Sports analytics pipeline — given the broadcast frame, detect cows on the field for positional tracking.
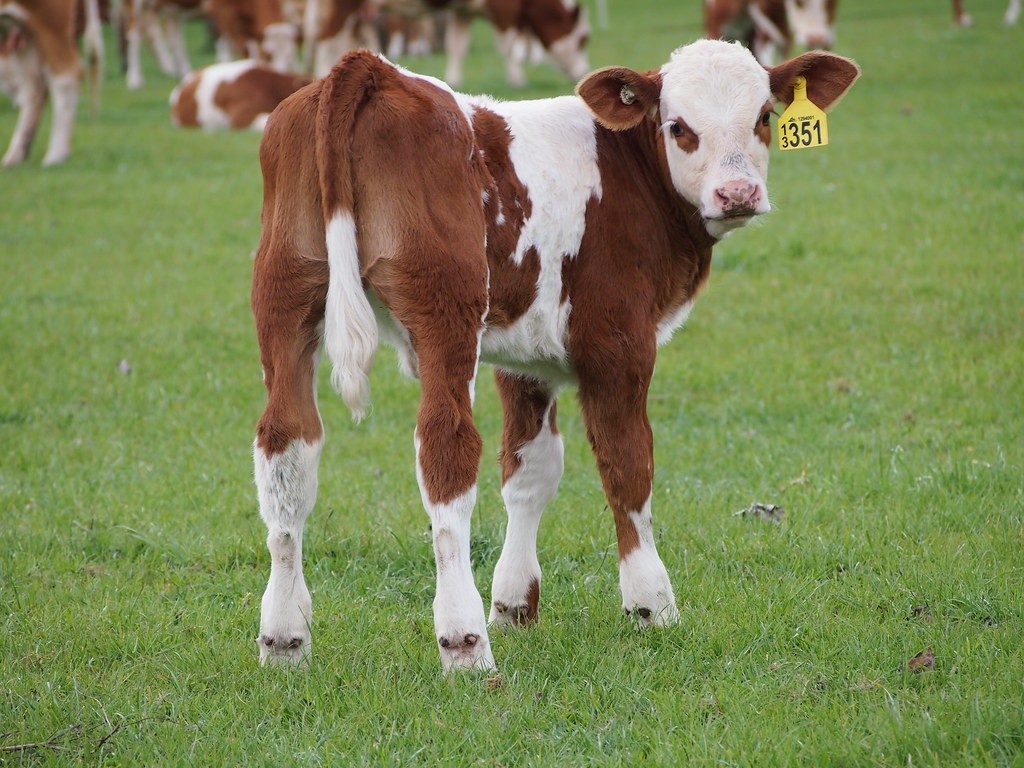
[695,0,841,68]
[251,35,859,690]
[0,1,596,165]
[953,1,1023,29]
